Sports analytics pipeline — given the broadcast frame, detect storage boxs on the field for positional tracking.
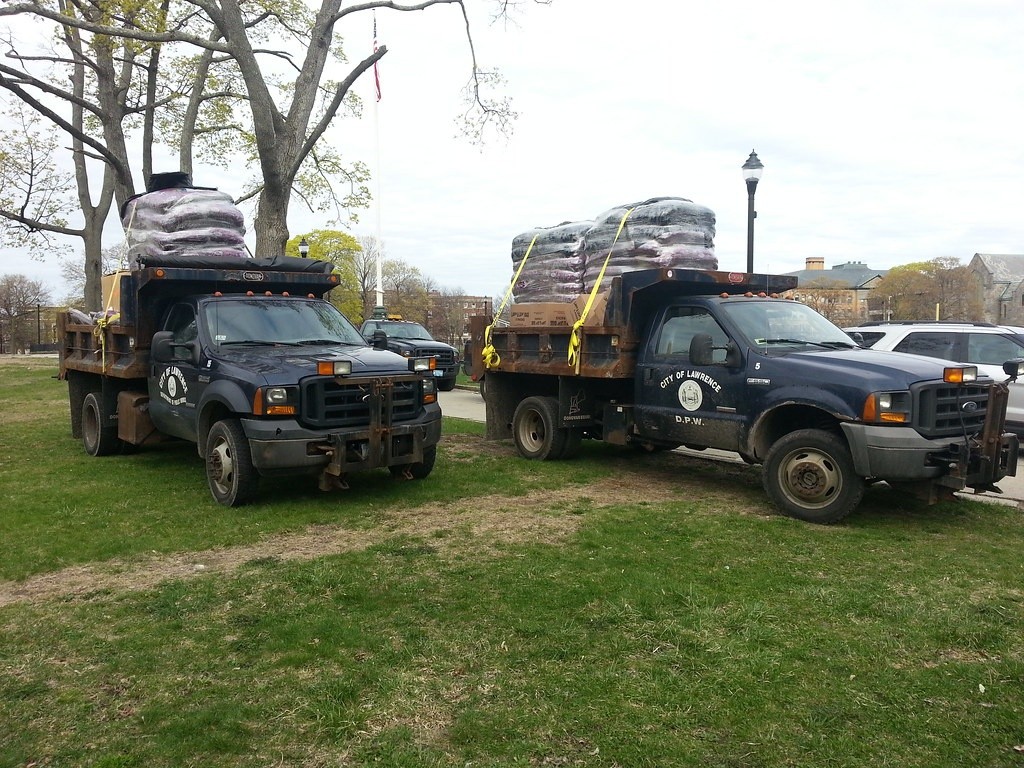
[511,304,572,327]
[112,269,131,312]
[102,272,115,311]
[594,286,612,325]
[564,294,603,325]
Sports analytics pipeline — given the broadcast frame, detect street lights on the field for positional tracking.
[741,148,765,272]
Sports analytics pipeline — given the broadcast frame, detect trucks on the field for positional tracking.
[458,268,1020,526]
[56,264,443,508]
[359,315,461,391]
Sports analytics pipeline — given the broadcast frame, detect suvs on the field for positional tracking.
[839,318,1023,445]
